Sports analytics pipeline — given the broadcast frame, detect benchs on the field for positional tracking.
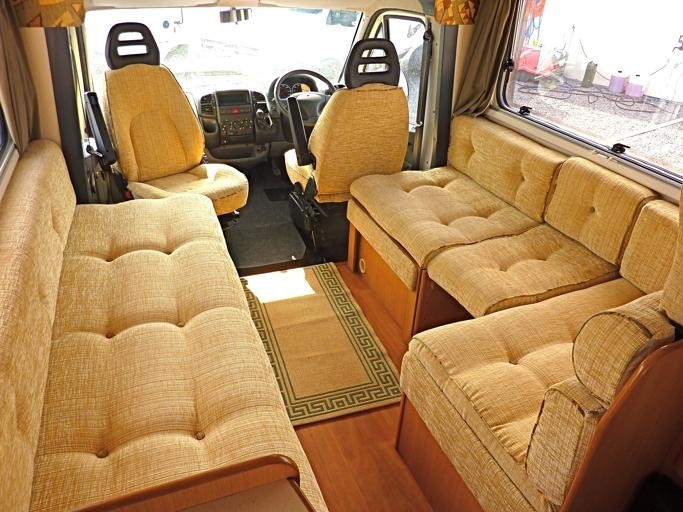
[344,115,660,369]
[0,138,327,512]
[393,191,683,511]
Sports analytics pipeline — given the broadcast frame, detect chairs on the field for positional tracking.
[81,22,249,214]
[284,38,409,256]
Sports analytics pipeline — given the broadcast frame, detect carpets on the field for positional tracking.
[238,261,404,427]
